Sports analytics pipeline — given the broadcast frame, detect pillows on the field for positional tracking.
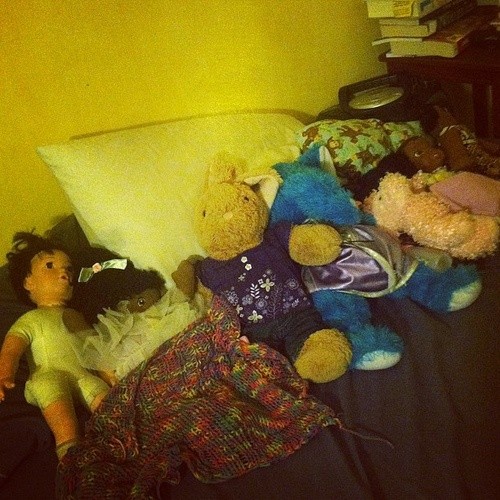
[34,112,309,293]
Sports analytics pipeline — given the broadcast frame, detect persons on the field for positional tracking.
[395,136,500,216]
[0,231,119,461]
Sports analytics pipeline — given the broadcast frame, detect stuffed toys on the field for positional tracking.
[68,144,500,384]
[420,103,500,180]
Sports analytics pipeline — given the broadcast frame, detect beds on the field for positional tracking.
[0,152,500,500]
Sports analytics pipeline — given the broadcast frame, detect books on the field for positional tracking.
[367,0,500,58]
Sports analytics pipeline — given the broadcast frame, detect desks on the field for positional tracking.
[379,5,500,139]
